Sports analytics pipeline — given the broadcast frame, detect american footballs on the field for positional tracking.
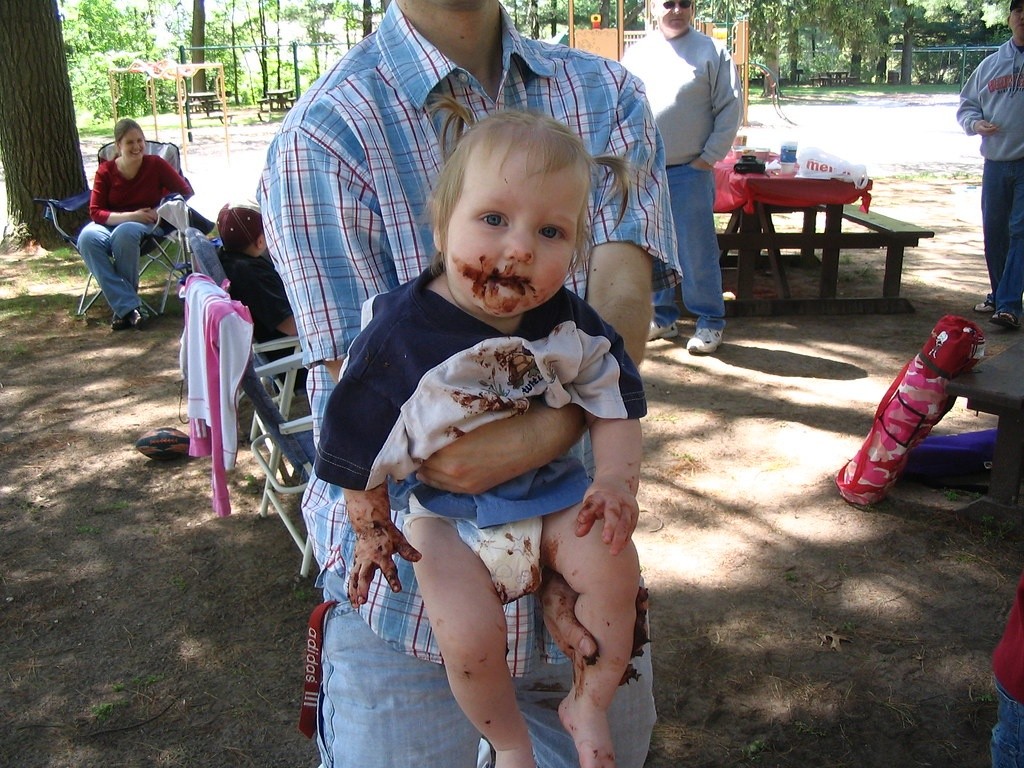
[136,427,190,461]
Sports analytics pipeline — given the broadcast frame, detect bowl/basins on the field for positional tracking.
[731,146,770,161]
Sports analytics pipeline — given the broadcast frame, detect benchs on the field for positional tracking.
[257,96,298,103]
[172,100,226,106]
[809,76,861,80]
[820,203,935,296]
[945,340,1024,505]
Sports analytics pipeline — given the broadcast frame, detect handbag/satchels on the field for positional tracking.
[793,147,868,189]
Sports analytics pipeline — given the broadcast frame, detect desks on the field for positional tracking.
[187,91,222,117]
[694,148,873,317]
[266,89,294,112]
[826,71,850,86]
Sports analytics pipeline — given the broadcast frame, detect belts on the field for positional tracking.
[666,164,688,170]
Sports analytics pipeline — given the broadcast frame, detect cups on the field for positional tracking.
[781,142,797,161]
[779,161,796,176]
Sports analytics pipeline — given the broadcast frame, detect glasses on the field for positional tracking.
[662,0,692,10]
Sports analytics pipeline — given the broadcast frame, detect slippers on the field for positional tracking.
[988,311,1022,328]
[973,301,996,312]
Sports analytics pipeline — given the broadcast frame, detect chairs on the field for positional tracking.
[181,228,298,441]
[32,140,198,317]
[180,274,320,577]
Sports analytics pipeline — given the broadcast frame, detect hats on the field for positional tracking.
[217,201,263,251]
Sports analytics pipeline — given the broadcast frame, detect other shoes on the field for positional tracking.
[110,317,126,330]
[128,307,150,329]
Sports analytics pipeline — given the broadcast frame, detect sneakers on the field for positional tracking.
[687,328,724,354]
[645,321,678,341]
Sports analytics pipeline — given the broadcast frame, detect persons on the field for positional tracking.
[78,118,193,331]
[990,569,1024,768]
[217,199,310,391]
[315,101,653,768]
[621,0,744,356]
[258,0,684,768]
[956,0,1024,330]
